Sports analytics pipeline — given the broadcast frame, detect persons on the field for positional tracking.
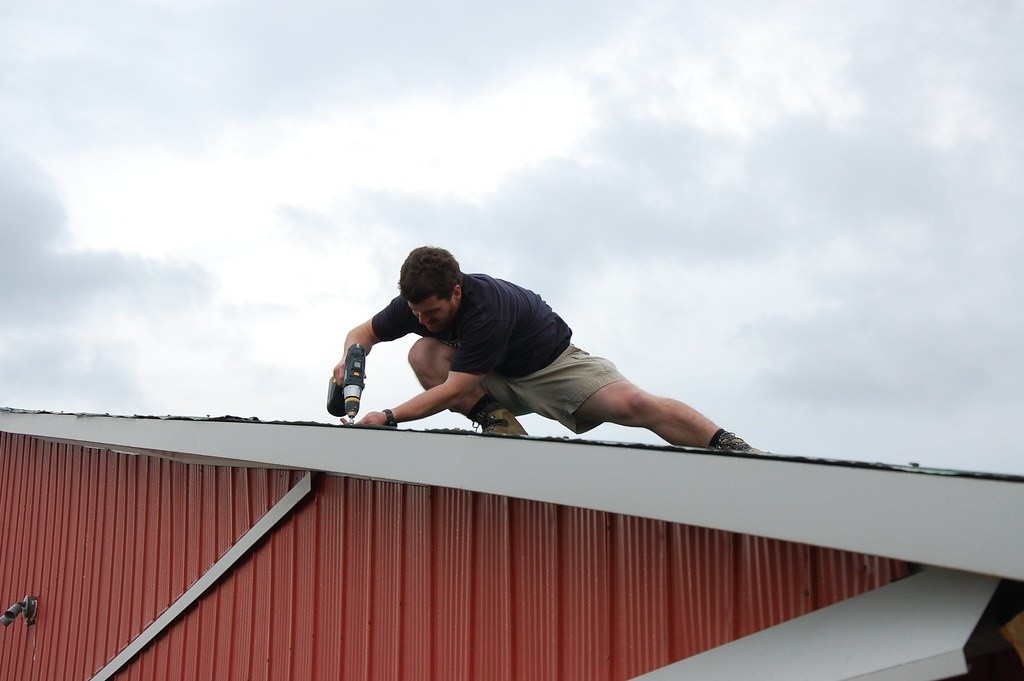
[335,247,765,455]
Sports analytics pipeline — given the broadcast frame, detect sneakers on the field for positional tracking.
[714,431,772,455]
[472,405,528,436]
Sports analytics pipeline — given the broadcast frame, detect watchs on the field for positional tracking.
[382,409,398,428]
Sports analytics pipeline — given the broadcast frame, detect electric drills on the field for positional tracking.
[326,342,367,427]
